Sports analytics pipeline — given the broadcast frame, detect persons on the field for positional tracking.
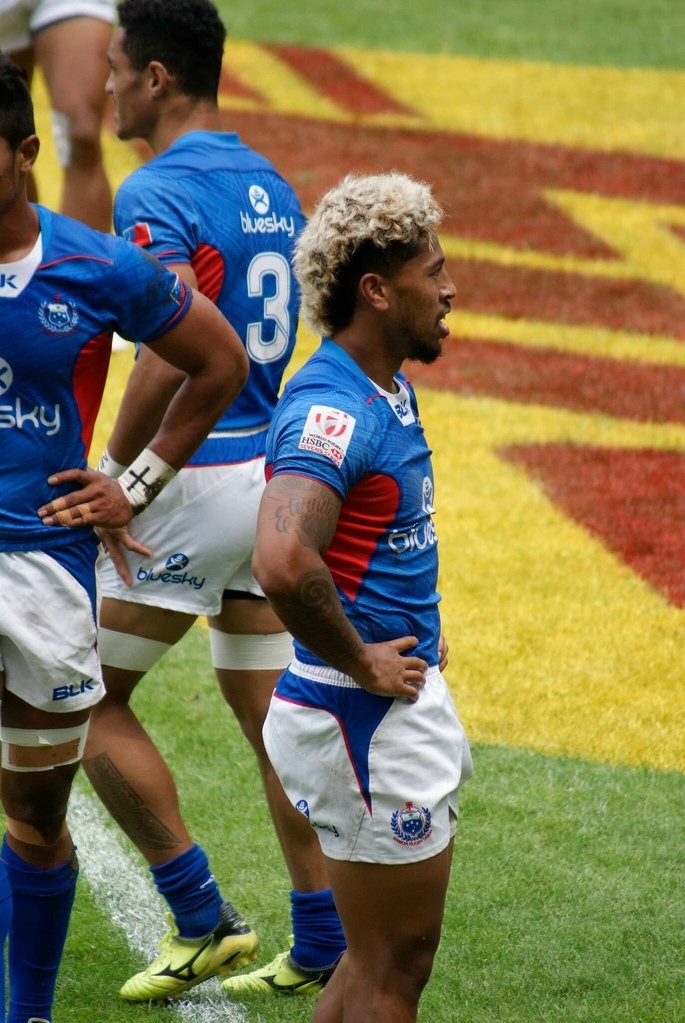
[0,56,251,1022]
[82,0,348,1004]
[0,0,123,234]
[251,170,475,1023]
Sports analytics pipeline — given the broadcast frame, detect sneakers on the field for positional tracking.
[221,932,325,994]
[119,900,258,1000]
